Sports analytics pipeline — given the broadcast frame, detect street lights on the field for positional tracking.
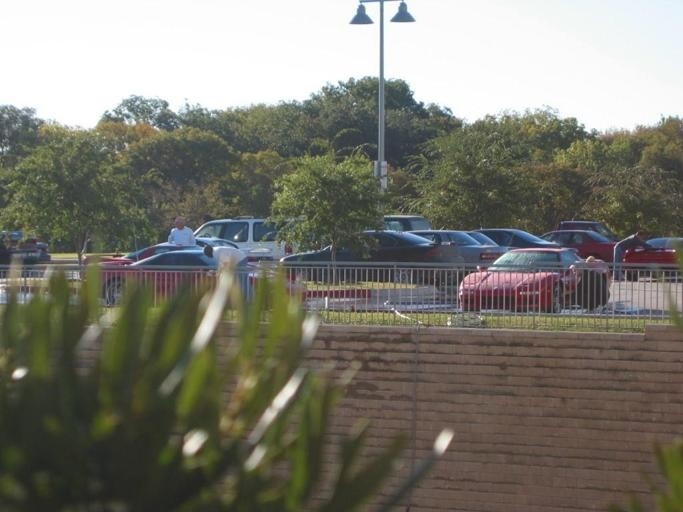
[350,0,415,190]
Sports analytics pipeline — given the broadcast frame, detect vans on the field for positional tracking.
[194,216,329,262]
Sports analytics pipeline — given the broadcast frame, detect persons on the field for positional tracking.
[570,255,611,287]
[168,217,196,248]
[612,229,655,281]
[0,229,50,280]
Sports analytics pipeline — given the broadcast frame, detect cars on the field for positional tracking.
[623,237,682,282]
[82,238,307,310]
[0,230,49,275]
